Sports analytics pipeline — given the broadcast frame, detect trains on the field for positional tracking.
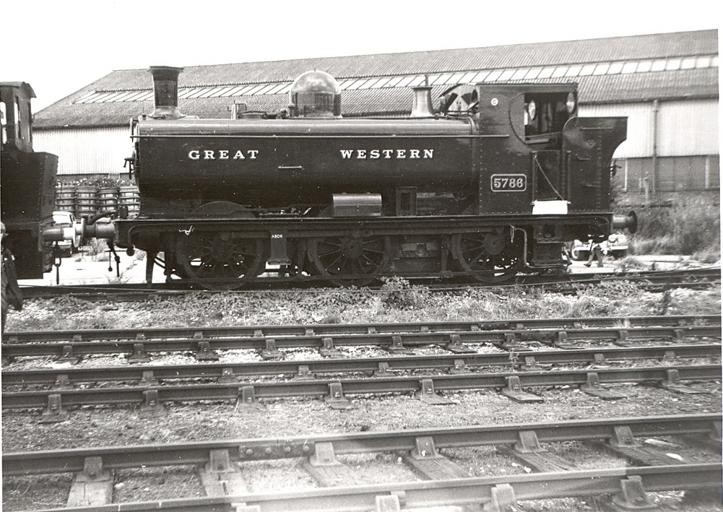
[0,54,643,294]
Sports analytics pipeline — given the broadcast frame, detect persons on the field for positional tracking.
[0,222,23,366]
[583,240,605,267]
